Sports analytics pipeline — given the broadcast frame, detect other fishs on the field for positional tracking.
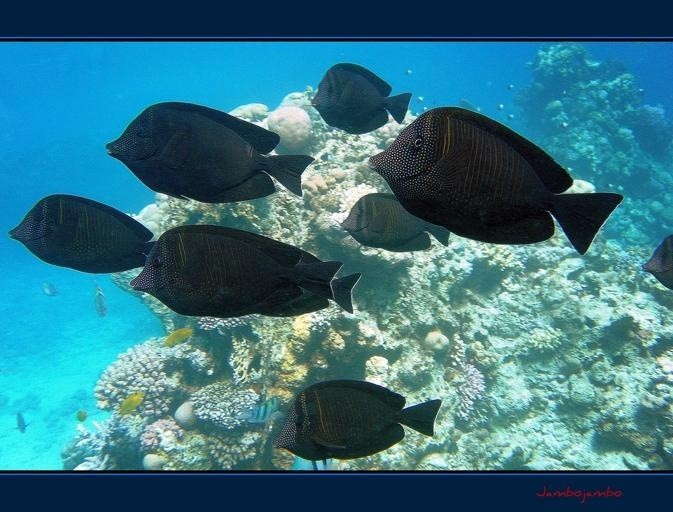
[368,97,625,256]
